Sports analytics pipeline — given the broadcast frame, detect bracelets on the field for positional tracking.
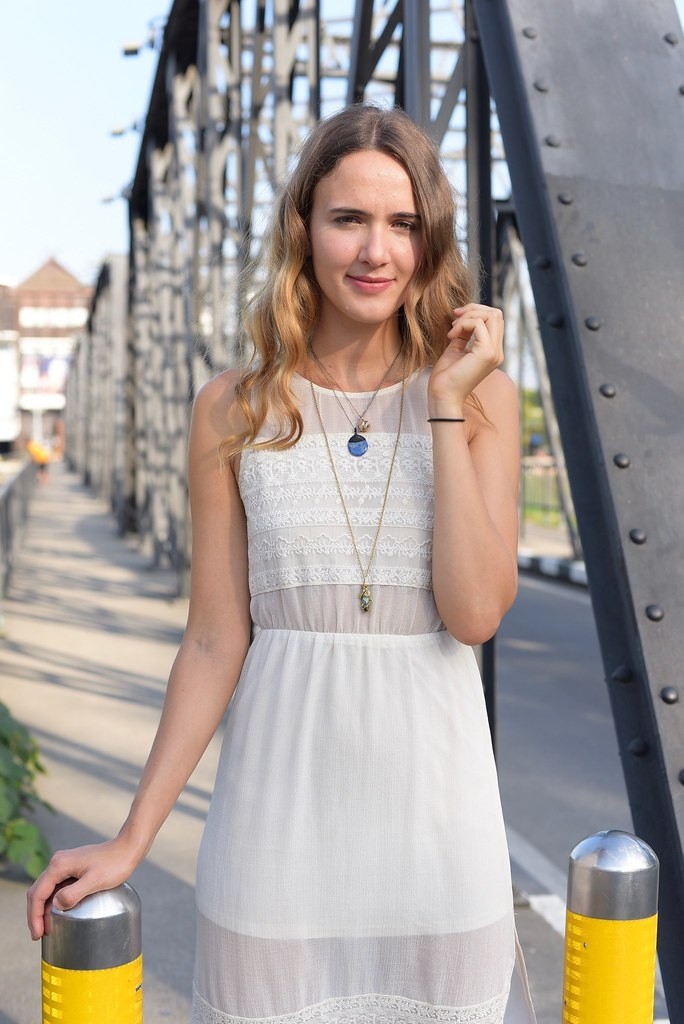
[427,417,465,422]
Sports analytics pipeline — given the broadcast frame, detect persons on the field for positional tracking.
[22,435,51,485]
[27,107,519,1024]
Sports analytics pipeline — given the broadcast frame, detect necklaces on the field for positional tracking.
[308,340,409,613]
